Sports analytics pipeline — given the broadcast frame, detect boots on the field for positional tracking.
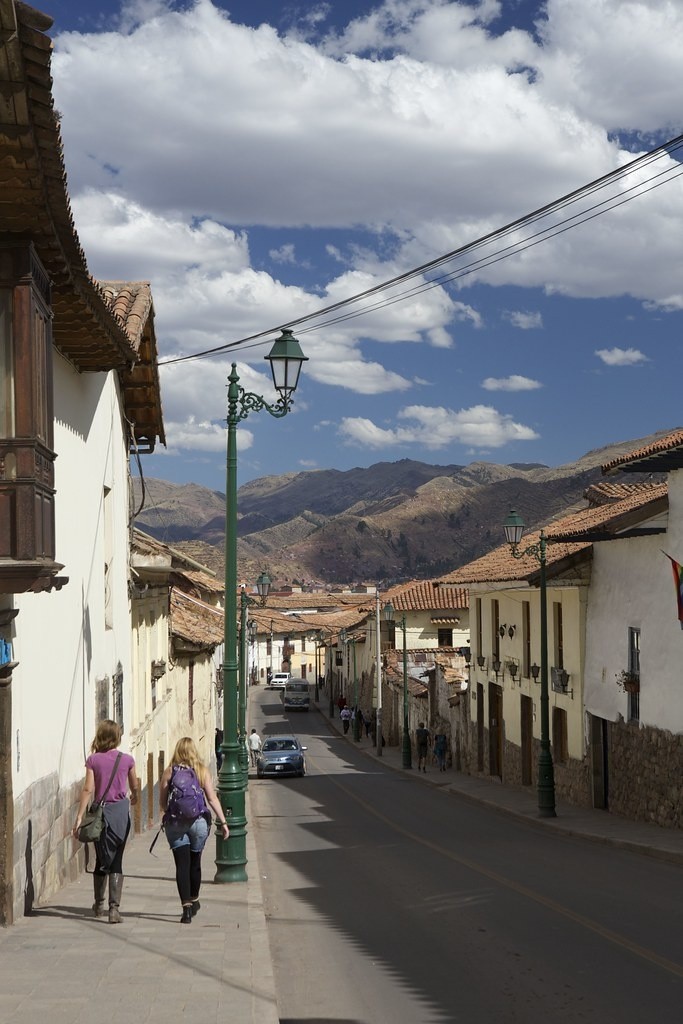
[108,872,126,924]
[91,874,106,917]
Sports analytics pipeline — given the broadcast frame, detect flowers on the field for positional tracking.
[616,671,638,693]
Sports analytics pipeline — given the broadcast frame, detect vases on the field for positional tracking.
[623,681,639,693]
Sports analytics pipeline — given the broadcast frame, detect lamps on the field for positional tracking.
[558,670,574,699]
[151,663,163,682]
[464,653,475,671]
[509,662,521,682]
[493,661,505,683]
[508,624,517,640]
[155,659,166,679]
[477,655,488,676]
[500,624,506,639]
[530,663,542,683]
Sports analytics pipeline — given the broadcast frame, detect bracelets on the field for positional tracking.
[221,821,227,826]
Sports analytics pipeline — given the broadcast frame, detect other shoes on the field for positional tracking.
[418,766,421,770]
[180,901,201,923]
[423,767,426,773]
[440,767,446,773]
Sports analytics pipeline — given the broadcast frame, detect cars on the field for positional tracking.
[270,673,292,689]
[254,736,308,778]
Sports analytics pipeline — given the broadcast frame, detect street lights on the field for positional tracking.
[384,601,413,770]
[319,630,336,720]
[246,621,258,699]
[238,571,271,784]
[216,327,310,880]
[311,632,318,700]
[340,627,359,742]
[504,509,555,819]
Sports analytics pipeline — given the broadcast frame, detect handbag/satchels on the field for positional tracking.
[79,802,108,842]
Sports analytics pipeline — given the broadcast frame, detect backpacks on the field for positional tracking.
[166,768,207,819]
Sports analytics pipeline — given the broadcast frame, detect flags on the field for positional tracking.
[667,556,683,627]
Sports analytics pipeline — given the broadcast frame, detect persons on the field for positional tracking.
[319,675,447,773]
[73,720,137,923]
[214,724,296,775]
[158,737,230,923]
[267,671,273,684]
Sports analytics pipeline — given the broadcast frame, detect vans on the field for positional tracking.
[284,677,310,711]
